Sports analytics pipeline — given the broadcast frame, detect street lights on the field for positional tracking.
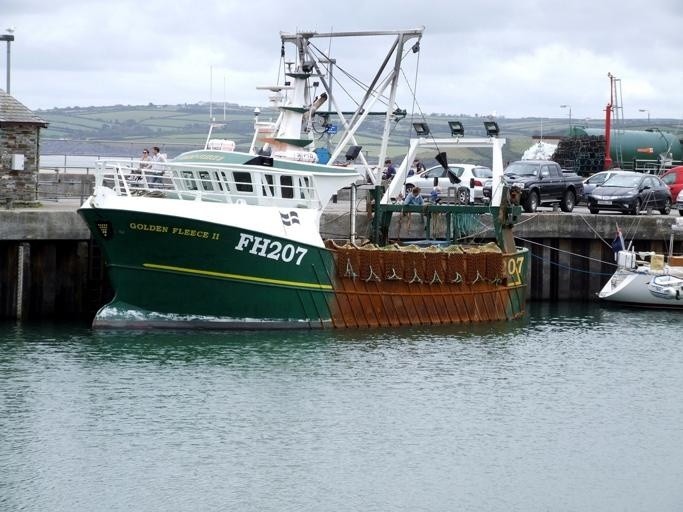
[558,104,572,136]
[642,125,669,154]
[637,108,650,130]
[0,27,15,94]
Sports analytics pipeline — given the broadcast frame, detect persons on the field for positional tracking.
[143,146,168,195]
[381,159,444,235]
[138,148,155,193]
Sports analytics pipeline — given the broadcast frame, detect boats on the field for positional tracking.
[85,23,536,337]
[593,243,682,310]
